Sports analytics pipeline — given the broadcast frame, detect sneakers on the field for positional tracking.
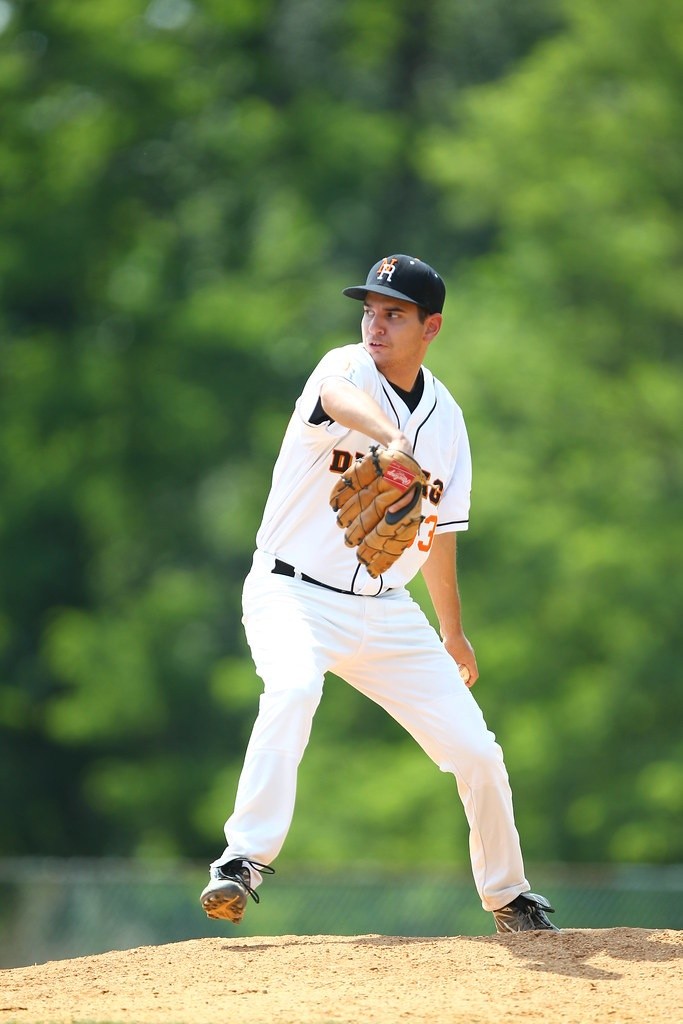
[200,858,275,924]
[492,893,560,933]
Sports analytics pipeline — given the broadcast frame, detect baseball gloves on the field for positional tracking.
[326,447,431,578]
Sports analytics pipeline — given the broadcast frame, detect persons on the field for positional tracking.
[197,254,558,934]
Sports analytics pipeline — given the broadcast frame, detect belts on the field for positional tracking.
[271,559,359,596]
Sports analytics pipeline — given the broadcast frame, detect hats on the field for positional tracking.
[342,254,446,314]
